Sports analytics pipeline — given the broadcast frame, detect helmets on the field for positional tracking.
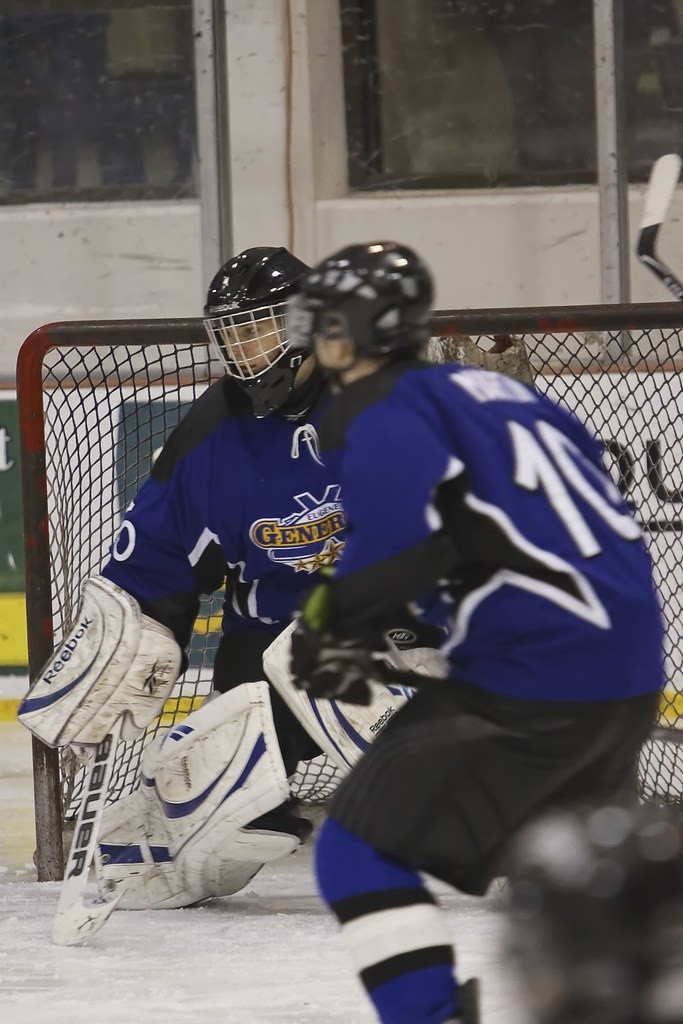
[202,247,314,418]
[286,242,433,356]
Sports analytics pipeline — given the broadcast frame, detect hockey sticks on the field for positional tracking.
[47,715,128,946]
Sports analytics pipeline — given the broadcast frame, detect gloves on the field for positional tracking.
[290,566,371,706]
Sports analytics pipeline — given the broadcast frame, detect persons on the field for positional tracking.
[500,797,683,1024]
[283,240,667,1024]
[19,246,406,910]
